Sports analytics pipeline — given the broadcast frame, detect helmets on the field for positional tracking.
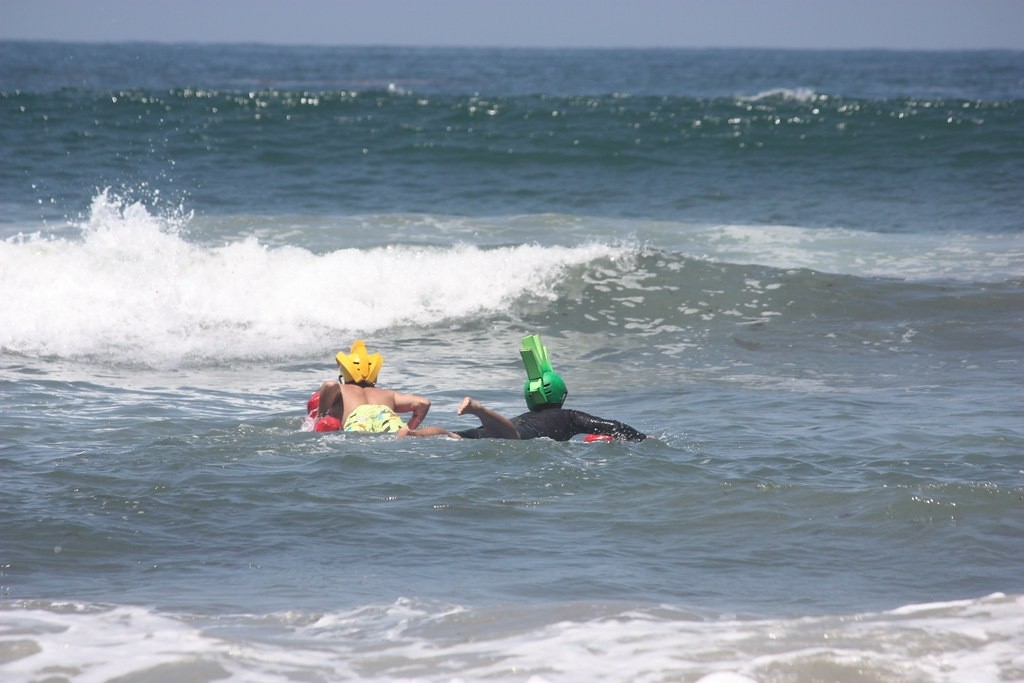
[521,334,567,410]
[334,341,383,386]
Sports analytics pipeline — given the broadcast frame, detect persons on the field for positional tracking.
[398,372,646,444]
[317,341,431,433]
